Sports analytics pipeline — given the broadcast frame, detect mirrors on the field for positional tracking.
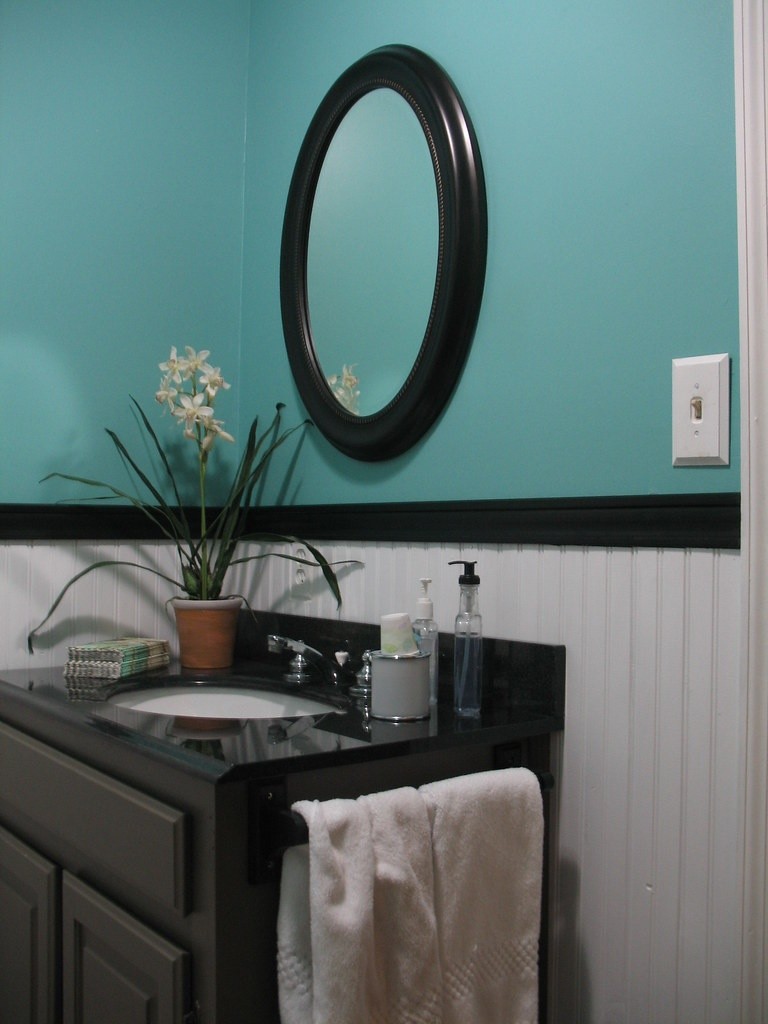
[278,44,488,464]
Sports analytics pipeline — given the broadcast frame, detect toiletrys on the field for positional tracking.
[411,576,438,707]
[447,558,484,718]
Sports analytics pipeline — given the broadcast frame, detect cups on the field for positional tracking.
[371,651,432,721]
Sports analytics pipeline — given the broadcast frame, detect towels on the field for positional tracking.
[274,764,546,1024]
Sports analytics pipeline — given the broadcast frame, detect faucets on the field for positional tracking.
[266,633,343,699]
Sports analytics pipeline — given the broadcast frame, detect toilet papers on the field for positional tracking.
[379,611,420,656]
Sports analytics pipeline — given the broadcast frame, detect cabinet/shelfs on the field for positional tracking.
[0,718,552,1024]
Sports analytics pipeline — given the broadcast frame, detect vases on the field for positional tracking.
[171,596,242,670]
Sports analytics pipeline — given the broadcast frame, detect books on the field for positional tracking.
[64,637,171,679]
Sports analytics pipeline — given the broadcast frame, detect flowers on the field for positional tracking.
[28,345,364,656]
[327,362,363,418]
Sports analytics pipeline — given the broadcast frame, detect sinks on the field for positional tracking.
[103,672,349,720]
